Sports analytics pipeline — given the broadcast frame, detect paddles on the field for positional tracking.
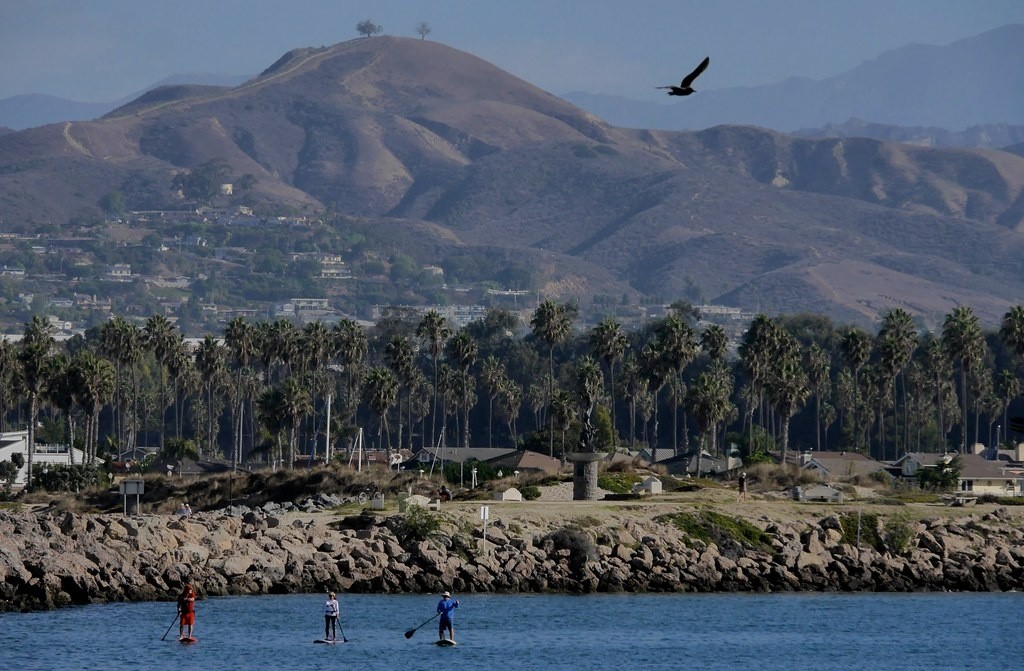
[162,614,179,641]
[333,606,349,642]
[404,602,457,639]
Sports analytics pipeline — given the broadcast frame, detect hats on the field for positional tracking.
[330,592,335,595]
[441,592,451,598]
[742,472,746,476]
[185,584,193,589]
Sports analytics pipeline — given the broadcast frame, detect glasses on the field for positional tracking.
[331,595,334,596]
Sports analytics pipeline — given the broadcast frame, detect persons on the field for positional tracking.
[738,472,746,502]
[437,591,459,640]
[323,592,339,641]
[437,486,452,503]
[179,503,191,518]
[177,585,195,641]
